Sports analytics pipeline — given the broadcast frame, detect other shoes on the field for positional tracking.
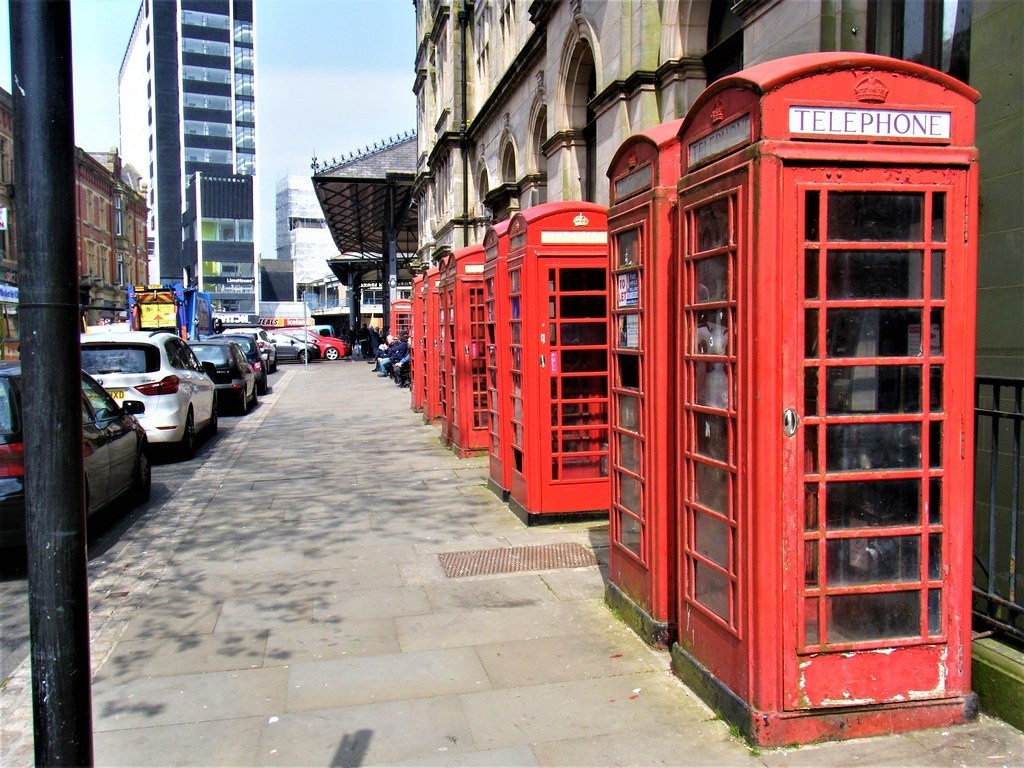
[367,360,375,364]
[372,367,378,372]
[362,354,366,360]
[377,372,387,377]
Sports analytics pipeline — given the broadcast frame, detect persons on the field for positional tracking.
[337,323,381,359]
[368,336,411,387]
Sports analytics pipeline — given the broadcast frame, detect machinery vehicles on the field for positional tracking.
[126,282,220,341]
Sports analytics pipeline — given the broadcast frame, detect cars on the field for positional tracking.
[222,326,277,374]
[276,326,352,361]
[0,358,153,545]
[266,330,320,364]
[183,338,260,414]
[205,333,271,395]
[80,330,220,458]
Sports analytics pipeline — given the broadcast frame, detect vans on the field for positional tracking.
[301,325,335,337]
